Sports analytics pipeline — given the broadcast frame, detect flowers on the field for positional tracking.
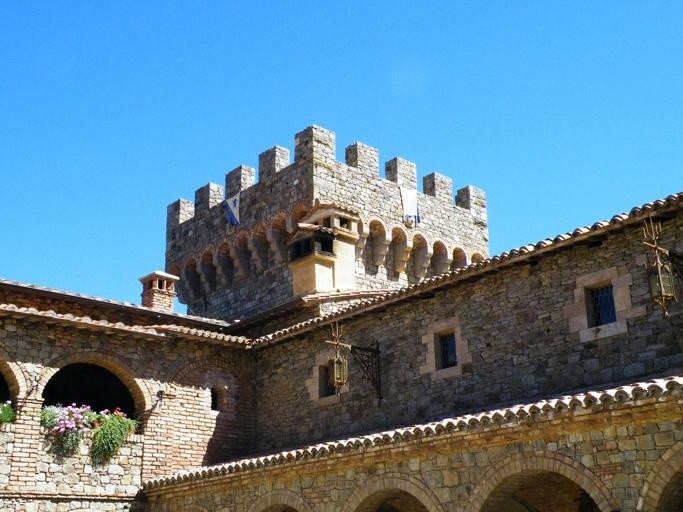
[41,402,96,456]
[99,407,127,421]
[0,400,17,424]
[225,384,240,398]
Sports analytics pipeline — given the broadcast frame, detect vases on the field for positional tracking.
[94,420,134,435]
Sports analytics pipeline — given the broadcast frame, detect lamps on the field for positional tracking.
[642,209,683,318]
[324,320,382,399]
